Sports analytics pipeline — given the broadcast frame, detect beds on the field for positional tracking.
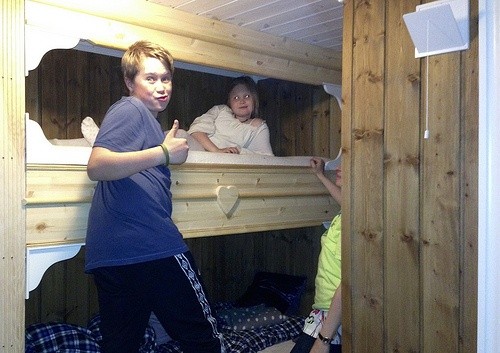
[24,112,343,353]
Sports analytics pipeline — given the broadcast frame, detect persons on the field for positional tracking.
[81,75,275,157]
[290,156,342,353]
[84,39,227,353]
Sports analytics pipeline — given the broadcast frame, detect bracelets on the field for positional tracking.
[159,144,169,166]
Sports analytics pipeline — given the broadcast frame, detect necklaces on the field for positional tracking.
[235,113,252,123]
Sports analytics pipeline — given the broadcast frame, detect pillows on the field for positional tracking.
[220,304,289,332]
[254,270,307,316]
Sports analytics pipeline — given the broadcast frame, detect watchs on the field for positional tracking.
[318,333,331,345]
